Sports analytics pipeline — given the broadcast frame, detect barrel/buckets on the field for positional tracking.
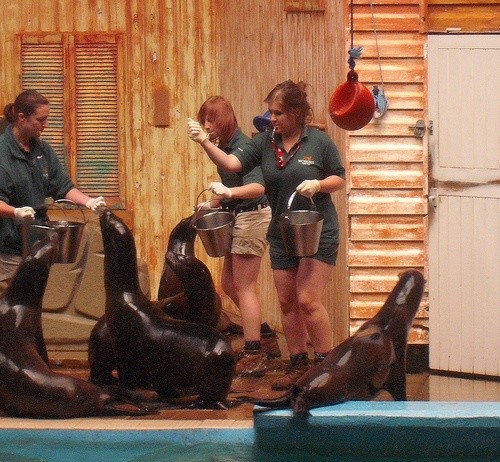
[43,199,86,264]
[21,204,69,264]
[278,190,325,256]
[194,188,229,221]
[191,199,235,257]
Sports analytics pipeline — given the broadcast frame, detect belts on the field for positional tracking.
[235,199,269,214]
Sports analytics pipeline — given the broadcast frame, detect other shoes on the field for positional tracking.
[272,351,332,391]
[234,354,266,377]
[267,357,292,370]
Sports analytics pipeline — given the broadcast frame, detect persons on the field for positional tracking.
[0,90,108,372]
[187,79,346,390]
[197,96,283,376]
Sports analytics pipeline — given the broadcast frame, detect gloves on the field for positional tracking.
[197,200,222,210]
[187,117,206,144]
[14,206,36,225]
[86,196,107,211]
[208,182,232,199]
[296,178,320,198]
